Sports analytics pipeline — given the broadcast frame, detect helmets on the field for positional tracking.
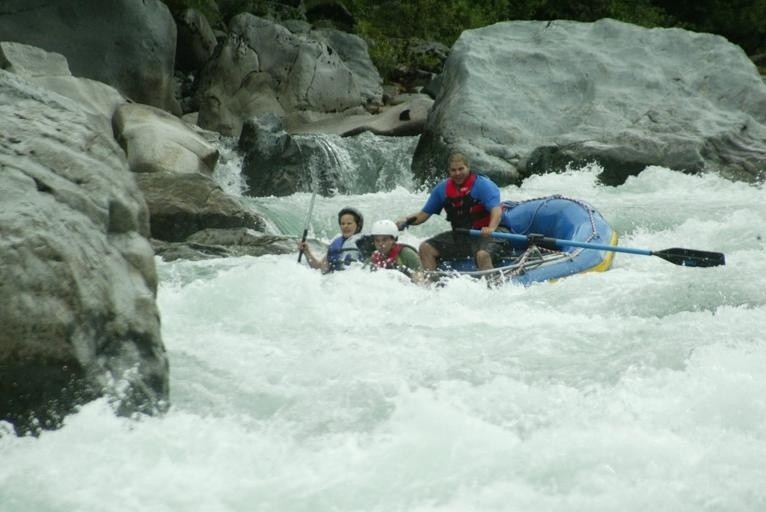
[338,206,365,234]
[368,217,399,239]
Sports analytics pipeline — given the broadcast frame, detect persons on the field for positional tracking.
[397,151,512,283]
[298,207,369,275]
[365,218,426,286]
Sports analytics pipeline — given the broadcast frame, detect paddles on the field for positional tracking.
[297,183,316,265]
[453,226,725,267]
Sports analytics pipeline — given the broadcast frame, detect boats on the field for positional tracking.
[438,194,619,286]
